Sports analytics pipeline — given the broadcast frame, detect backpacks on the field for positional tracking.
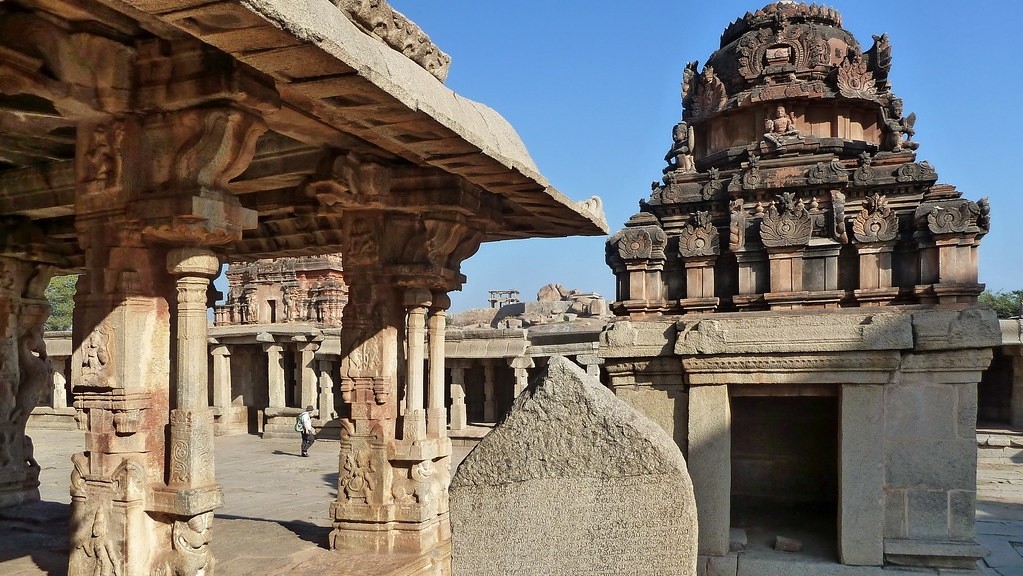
[294,413,304,431]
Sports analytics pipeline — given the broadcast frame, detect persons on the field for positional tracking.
[301,405,315,457]
[662,122,694,174]
[763,107,800,148]
[884,100,919,150]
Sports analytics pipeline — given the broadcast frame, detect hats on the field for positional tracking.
[306,406,315,411]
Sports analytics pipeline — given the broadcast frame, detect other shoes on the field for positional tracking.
[302,452,309,457]
